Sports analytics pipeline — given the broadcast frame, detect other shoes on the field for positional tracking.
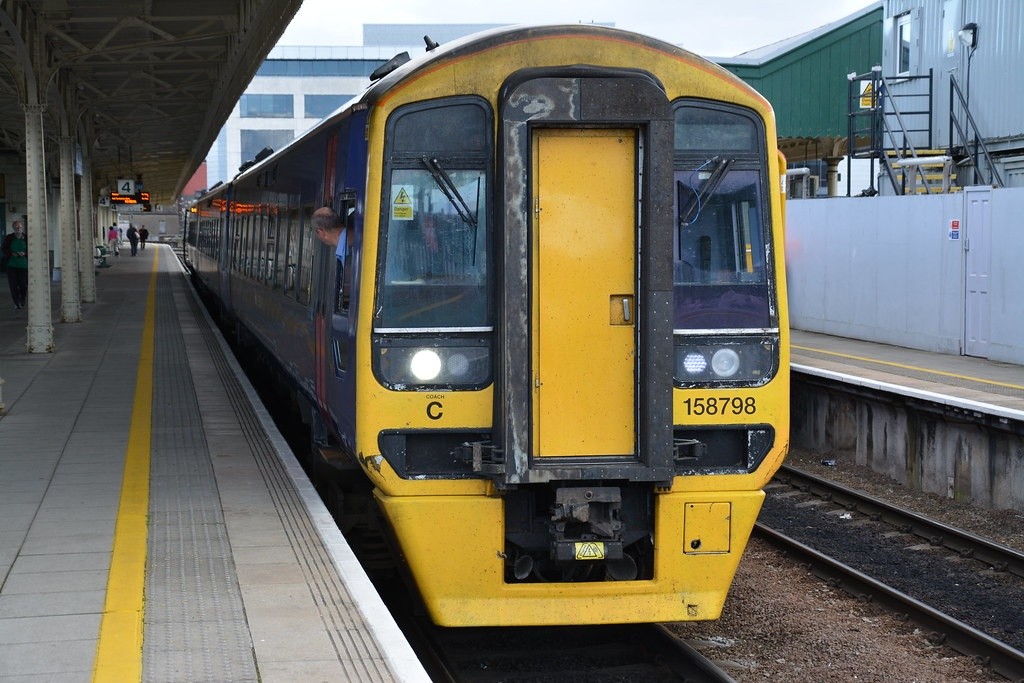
[15,304,23,311]
[141,247,145,250]
[114,252,119,256]
[132,254,136,256]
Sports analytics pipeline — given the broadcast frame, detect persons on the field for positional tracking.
[2,220,28,309]
[310,207,353,316]
[139,225,149,250]
[107,222,122,256]
[127,224,138,256]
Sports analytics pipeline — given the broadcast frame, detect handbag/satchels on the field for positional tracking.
[135,232,141,238]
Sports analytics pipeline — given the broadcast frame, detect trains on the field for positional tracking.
[179,22,790,629]
